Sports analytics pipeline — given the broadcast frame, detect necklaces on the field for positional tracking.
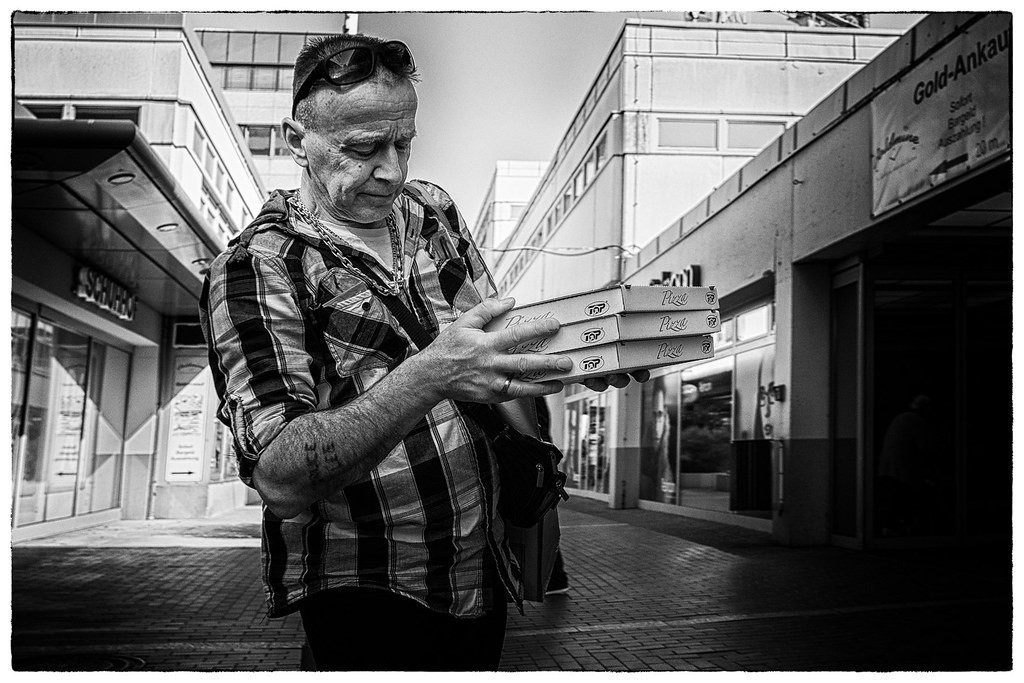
[293,188,405,297]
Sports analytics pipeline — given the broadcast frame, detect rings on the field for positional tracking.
[499,375,513,396]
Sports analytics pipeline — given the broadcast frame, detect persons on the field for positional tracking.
[199,32,570,671]
[641,351,780,516]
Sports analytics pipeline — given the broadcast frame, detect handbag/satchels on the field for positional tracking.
[493,420,570,603]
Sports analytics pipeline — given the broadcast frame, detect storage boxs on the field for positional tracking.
[478,285,723,384]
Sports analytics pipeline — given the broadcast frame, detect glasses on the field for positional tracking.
[291,39,416,119]
